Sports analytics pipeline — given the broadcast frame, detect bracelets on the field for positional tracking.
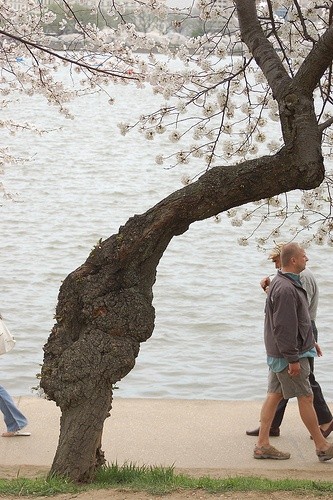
[262,284,269,292]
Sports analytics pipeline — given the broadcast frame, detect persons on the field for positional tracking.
[253,242,333,461]
[0,316,32,437]
[245,242,333,440]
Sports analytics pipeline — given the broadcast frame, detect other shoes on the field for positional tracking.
[310,421,333,440]
[246,426,279,436]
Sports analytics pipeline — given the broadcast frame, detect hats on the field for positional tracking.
[268,242,289,259]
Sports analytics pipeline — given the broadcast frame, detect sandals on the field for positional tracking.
[316,444,333,462]
[253,443,290,460]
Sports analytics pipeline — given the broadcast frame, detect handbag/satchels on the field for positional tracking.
[0,318,16,355]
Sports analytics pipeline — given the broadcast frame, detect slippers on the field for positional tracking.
[1,431,31,436]
